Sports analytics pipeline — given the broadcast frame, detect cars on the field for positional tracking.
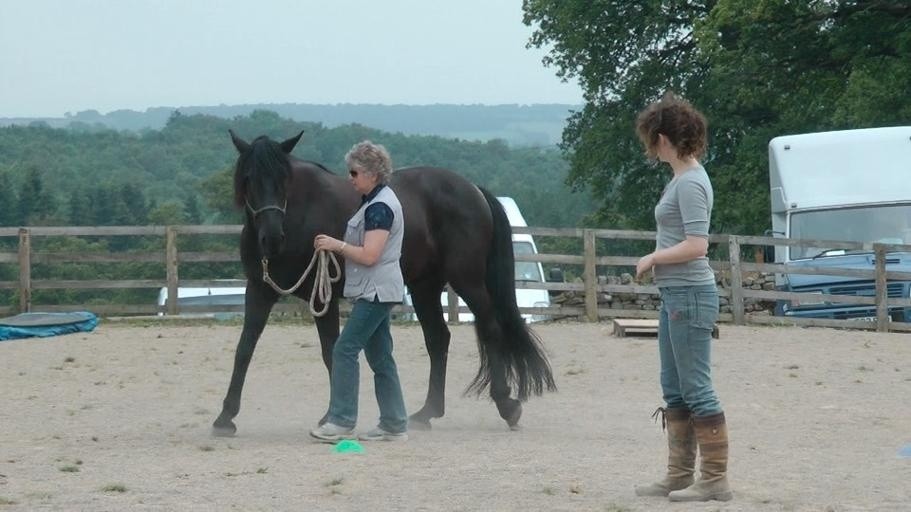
[157,275,261,321]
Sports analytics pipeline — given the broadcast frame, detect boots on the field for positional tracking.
[635,407,735,504]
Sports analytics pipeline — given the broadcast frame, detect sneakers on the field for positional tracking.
[310,421,356,441]
[357,424,410,443]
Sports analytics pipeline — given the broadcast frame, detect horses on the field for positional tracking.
[211,129,558,435]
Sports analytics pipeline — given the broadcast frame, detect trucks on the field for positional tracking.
[756,124,911,333]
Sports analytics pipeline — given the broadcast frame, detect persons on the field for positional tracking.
[635,93,735,503]
[308,141,410,442]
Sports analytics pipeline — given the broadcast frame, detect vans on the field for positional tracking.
[396,194,566,331]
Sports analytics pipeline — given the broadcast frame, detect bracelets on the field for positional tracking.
[336,239,347,256]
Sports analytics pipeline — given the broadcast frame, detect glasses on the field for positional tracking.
[349,169,368,178]
